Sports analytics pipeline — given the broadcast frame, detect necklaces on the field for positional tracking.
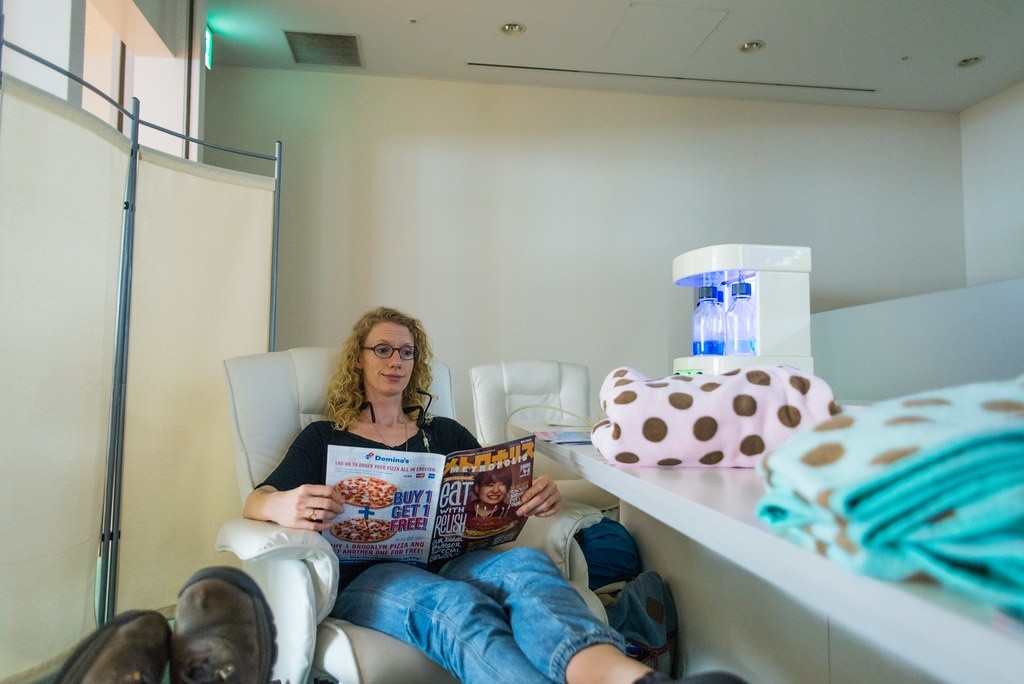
[361,410,408,452]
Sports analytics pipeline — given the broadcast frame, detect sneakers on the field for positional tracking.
[169,567,277,684]
[55,609,172,684]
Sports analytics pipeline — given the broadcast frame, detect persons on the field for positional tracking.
[51,565,279,684]
[242,306,748,684]
[464,468,512,518]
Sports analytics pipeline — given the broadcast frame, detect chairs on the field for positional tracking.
[468,358,620,520]
[215,344,609,684]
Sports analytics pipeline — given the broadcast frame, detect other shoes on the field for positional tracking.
[630,669,744,684]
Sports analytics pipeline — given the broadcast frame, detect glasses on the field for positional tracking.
[359,343,420,360]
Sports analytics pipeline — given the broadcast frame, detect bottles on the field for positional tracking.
[691,286,724,355]
[724,283,756,356]
[713,291,725,321]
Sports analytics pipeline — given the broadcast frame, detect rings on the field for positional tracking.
[309,509,317,520]
